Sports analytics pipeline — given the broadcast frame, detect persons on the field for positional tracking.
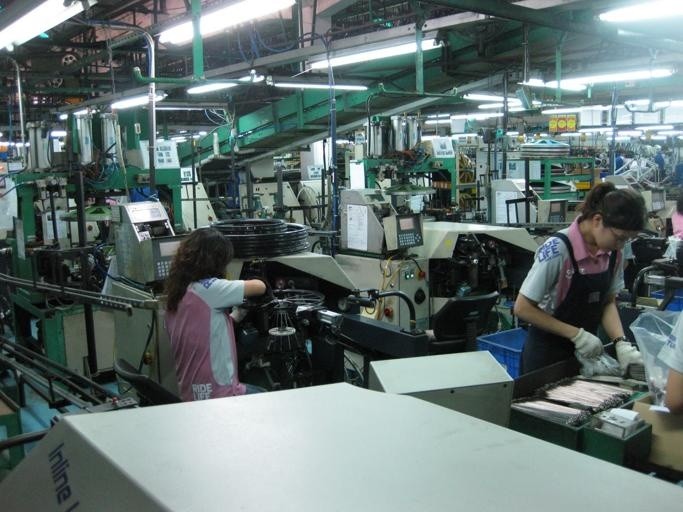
[656,310,683,415]
[73,191,119,240]
[510,179,656,379]
[162,225,269,399]
[670,192,682,242]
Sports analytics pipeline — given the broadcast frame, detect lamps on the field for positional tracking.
[185,73,264,94]
[303,30,450,68]
[110,90,168,110]
[263,75,371,91]
[154,1,296,47]
[0,1,98,51]
[451,0,683,141]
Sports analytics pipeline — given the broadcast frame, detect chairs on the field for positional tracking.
[111,357,187,405]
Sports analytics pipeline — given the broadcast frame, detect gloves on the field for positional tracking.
[614,340,644,376]
[228,306,249,322]
[569,327,604,358]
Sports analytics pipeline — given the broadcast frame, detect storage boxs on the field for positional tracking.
[475,327,528,378]
[650,289,683,311]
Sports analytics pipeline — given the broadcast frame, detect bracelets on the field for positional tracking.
[613,334,630,344]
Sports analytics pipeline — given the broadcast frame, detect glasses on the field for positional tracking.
[608,227,641,251]
